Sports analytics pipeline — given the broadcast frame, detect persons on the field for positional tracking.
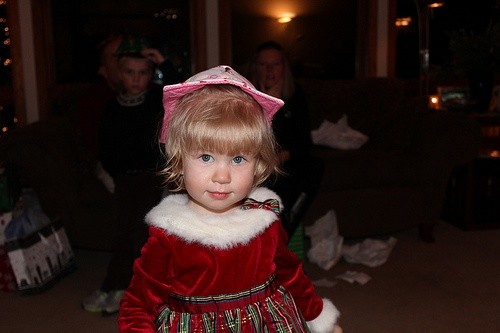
[80,33,186,314]
[116,65,343,333]
[244,41,317,247]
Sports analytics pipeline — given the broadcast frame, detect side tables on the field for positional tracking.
[463,113,500,232]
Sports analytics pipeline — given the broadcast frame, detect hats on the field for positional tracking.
[113,35,159,59]
[159,65,286,145]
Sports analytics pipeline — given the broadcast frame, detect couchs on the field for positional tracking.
[0,76,482,256]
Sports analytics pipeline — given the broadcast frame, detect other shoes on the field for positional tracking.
[100,290,125,313]
[80,288,107,313]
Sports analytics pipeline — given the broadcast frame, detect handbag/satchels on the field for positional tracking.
[8,217,79,297]
[441,156,500,231]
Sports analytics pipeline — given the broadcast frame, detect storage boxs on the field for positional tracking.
[5,215,80,296]
